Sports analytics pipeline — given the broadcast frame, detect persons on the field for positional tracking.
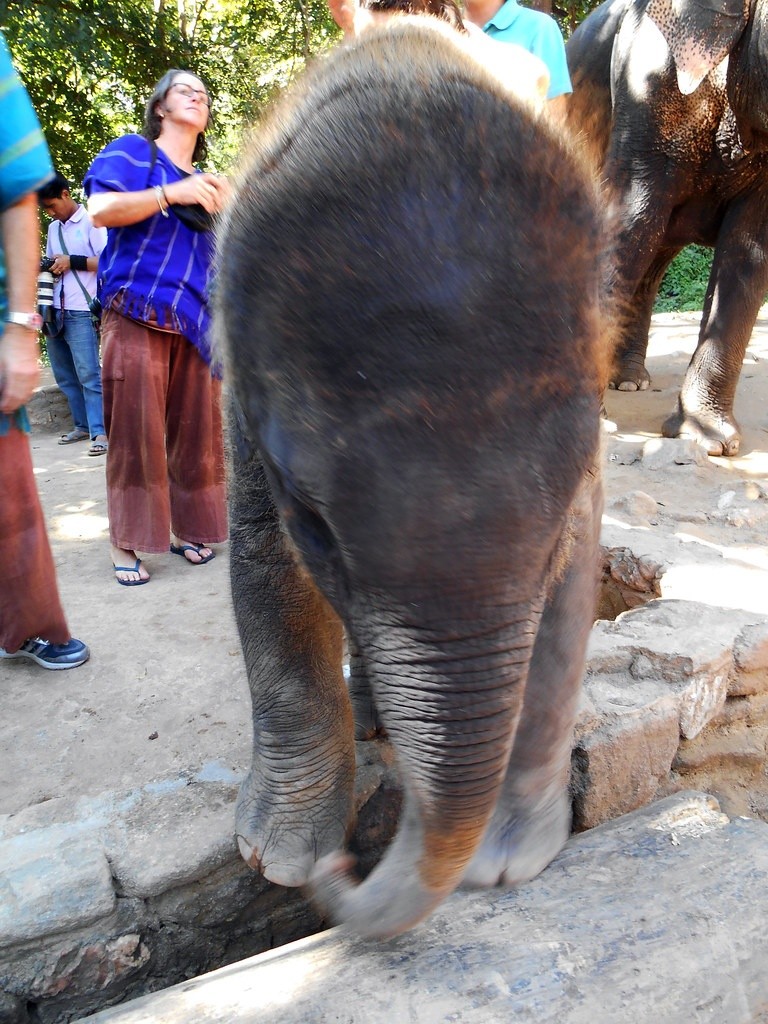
[34,176,109,456]
[323,0,573,127]
[0,35,90,671]
[82,67,231,586]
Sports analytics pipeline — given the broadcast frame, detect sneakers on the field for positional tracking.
[0,634,90,670]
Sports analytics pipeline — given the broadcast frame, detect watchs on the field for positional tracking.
[6,312,42,331]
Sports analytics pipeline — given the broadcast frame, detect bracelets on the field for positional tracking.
[69,254,89,272]
[155,186,170,218]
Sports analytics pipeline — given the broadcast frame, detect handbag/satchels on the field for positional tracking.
[88,295,103,319]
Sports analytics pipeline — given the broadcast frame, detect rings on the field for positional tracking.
[59,270,61,273]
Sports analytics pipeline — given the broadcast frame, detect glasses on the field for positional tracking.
[167,83,213,107]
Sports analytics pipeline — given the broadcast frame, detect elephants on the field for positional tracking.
[196,19,618,950]
[551,0,768,468]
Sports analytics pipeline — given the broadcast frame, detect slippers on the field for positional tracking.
[170,542,216,564]
[88,440,109,456]
[111,557,150,585]
[58,429,90,444]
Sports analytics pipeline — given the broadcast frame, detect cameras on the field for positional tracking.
[37,257,62,322]
